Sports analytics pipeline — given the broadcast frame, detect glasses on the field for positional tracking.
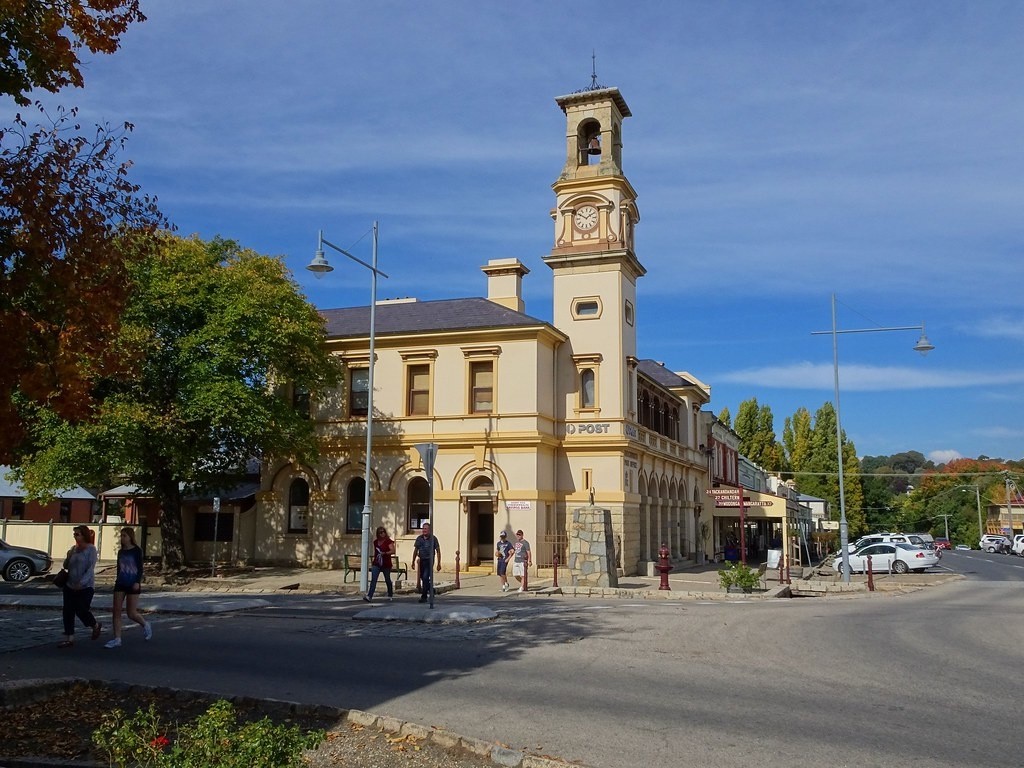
[120,534,128,537]
[380,531,386,534]
[517,534,521,535]
[74,533,80,536]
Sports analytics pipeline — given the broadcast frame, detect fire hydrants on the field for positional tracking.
[654,545,674,590]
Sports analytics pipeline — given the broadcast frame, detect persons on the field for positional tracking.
[751,534,780,560]
[58,526,102,649]
[104,528,152,648]
[411,523,441,602]
[497,530,515,592]
[511,530,532,592]
[363,526,395,603]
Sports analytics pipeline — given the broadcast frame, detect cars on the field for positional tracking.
[837,533,943,559]
[0,537,54,581]
[832,542,939,574]
[934,537,952,550]
[979,534,1024,557]
[955,544,971,551]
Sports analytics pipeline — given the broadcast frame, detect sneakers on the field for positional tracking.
[104,640,121,648]
[144,622,152,640]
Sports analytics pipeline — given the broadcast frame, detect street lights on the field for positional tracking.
[305,229,390,592]
[962,488,983,540]
[931,513,954,540]
[811,322,936,583]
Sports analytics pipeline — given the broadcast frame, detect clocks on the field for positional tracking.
[571,201,599,240]
[626,213,631,245]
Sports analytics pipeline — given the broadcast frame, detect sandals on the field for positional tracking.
[92,622,102,640]
[57,641,74,648]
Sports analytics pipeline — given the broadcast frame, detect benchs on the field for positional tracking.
[344,555,408,583]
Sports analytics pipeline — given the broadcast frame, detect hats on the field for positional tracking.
[500,531,507,536]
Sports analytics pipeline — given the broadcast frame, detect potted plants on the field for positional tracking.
[787,529,801,540]
[716,559,760,594]
[807,531,838,542]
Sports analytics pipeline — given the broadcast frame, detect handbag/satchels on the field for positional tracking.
[373,554,382,568]
[53,568,69,588]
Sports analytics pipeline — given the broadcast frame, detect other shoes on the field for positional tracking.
[518,586,524,592]
[419,598,427,602]
[387,597,392,601]
[505,582,509,591]
[363,596,372,603]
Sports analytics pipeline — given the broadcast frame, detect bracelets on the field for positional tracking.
[529,561,531,562]
[385,552,386,555]
